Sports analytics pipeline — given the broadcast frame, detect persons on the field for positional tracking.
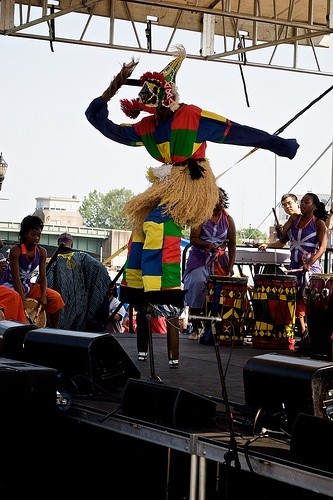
[109,282,188,333]
[84,50,300,367]
[257,193,333,336]
[37,232,111,333]
[183,188,236,340]
[0,215,66,329]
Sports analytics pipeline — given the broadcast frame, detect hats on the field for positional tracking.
[140,49,186,108]
[58,233,74,247]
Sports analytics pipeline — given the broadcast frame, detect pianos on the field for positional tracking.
[225,246,291,277]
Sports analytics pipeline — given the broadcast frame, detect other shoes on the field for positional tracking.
[188,332,199,340]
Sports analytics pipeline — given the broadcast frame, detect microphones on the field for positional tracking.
[188,315,222,323]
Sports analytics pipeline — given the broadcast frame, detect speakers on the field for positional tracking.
[122,378,217,430]
[291,414,333,471]
[0,320,37,361]
[20,329,141,399]
[241,354,333,433]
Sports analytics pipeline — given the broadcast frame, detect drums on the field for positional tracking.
[0,298,47,328]
[205,274,248,344]
[307,274,333,362]
[251,274,297,351]
